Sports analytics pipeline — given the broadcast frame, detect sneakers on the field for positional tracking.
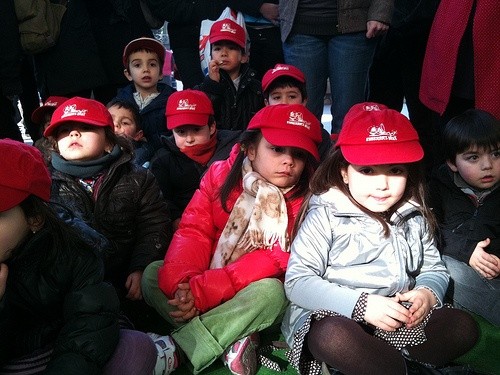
[146,331,181,375]
[222,333,262,375]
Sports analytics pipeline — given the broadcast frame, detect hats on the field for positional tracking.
[30,96,70,124]
[45,97,116,137]
[330,103,425,165]
[166,89,215,131]
[0,136,53,210]
[261,63,305,93]
[246,104,325,162]
[121,37,166,68]
[209,18,246,48]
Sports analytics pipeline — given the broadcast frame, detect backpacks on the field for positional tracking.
[11,0,67,56]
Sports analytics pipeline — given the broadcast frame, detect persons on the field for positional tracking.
[142,104,324,375]
[260,64,333,165]
[0,138,157,375]
[37,94,176,334]
[370,0,458,161]
[145,88,244,225]
[419,106,500,327]
[418,0,500,158]
[278,0,397,134]
[1,0,283,161]
[281,108,481,375]
